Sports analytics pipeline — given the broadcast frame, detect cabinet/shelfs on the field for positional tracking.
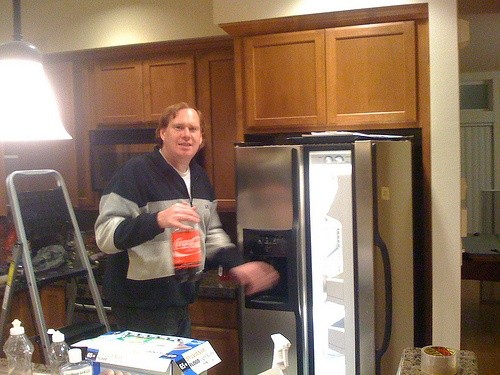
[0,3,431,375]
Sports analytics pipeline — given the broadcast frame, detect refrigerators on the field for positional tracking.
[236,132,419,375]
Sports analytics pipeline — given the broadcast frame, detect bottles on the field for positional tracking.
[172,226,206,303]
[3,319,34,375]
[42,328,93,375]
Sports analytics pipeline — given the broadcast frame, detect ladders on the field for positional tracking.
[0,168,113,367]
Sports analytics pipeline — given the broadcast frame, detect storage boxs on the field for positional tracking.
[71,330,222,375]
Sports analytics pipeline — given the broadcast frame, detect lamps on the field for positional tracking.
[0,0,72,143]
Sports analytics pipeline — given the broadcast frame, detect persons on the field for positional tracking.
[94,102,278,338]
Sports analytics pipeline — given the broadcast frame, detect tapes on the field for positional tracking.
[420,345,457,375]
